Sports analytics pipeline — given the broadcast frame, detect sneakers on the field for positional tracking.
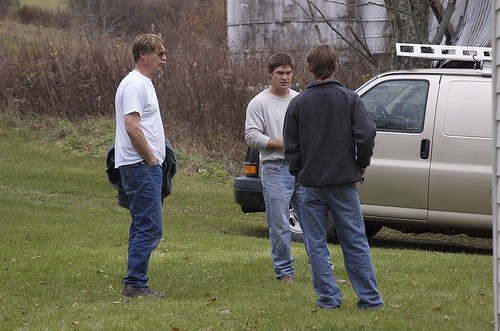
[148,286,164,297]
[124,285,161,298]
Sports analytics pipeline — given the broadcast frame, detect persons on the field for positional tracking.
[114,33,167,299]
[283,44,384,314]
[245,52,347,285]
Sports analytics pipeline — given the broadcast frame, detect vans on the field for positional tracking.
[235,43,492,241]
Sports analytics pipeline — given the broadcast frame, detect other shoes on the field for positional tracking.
[336,280,346,285]
[280,277,292,283]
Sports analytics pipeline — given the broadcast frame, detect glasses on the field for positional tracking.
[153,52,165,57]
